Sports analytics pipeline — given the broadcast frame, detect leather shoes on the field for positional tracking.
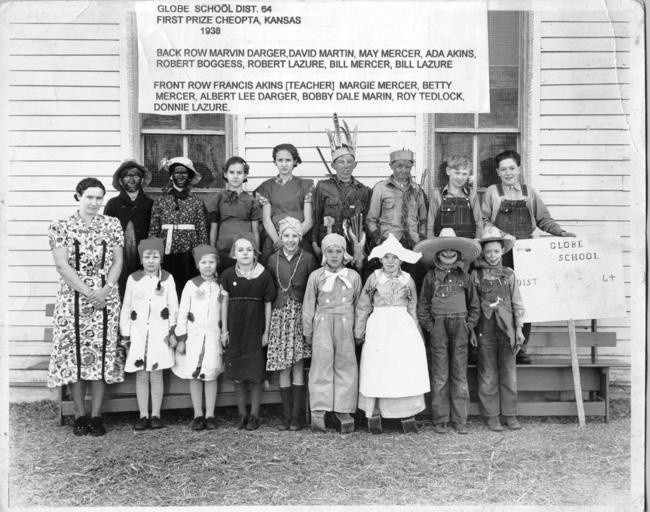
[206,416,217,430]
[149,416,163,428]
[245,415,259,430]
[234,415,246,429]
[134,416,148,429]
[192,416,206,430]
[90,416,105,435]
[73,415,89,437]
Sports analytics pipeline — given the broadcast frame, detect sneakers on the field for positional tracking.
[505,416,521,430]
[435,422,449,434]
[452,422,468,434]
[487,416,504,431]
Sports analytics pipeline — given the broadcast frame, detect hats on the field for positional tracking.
[479,223,516,256]
[367,233,423,265]
[192,244,220,269]
[163,156,202,186]
[138,237,165,264]
[389,148,413,164]
[112,160,152,190]
[324,114,358,162]
[414,227,482,266]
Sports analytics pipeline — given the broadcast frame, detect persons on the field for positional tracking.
[44,143,317,434]
[299,142,579,434]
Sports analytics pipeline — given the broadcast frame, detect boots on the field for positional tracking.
[278,386,291,430]
[289,383,305,431]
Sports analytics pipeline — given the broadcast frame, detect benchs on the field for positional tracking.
[41,300,617,429]
